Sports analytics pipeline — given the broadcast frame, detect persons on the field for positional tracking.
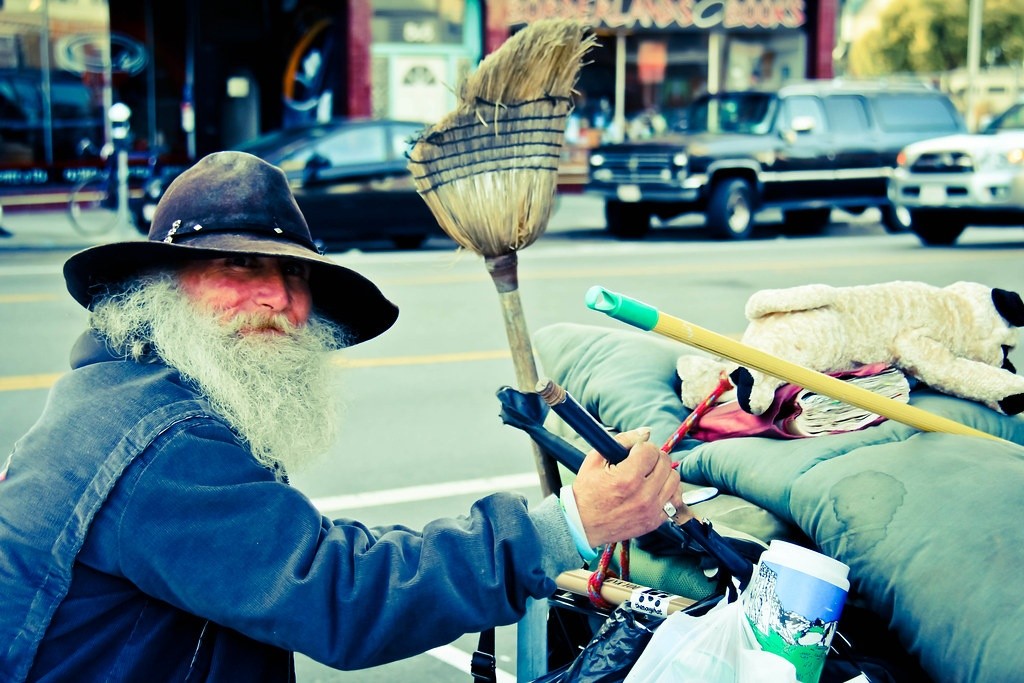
[0,151,683,683]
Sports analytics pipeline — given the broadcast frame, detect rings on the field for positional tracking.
[663,501,677,518]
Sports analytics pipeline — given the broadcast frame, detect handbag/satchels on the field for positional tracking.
[622,564,764,683]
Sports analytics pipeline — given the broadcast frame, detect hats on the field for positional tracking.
[64,150,400,351]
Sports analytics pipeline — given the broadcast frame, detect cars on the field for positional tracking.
[889,104,1024,247]
[139,121,450,255]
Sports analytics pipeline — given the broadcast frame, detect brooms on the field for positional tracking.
[404,17,602,500]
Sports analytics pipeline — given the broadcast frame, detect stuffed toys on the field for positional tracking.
[673,278,1024,417]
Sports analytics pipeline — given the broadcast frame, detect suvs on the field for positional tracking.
[582,84,971,240]
